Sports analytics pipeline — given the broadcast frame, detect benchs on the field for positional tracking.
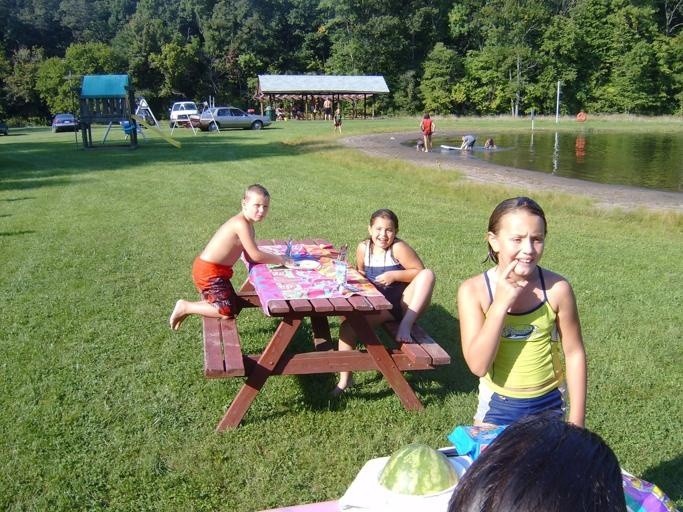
[203,316,245,378]
[383,318,452,367]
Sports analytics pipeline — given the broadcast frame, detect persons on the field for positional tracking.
[455,194,588,444]
[322,97,332,121]
[325,207,437,400]
[332,110,343,135]
[291,105,298,119]
[299,112,305,120]
[415,138,425,152]
[444,404,627,510]
[459,135,475,151]
[483,138,496,150]
[167,182,301,333]
[419,112,435,153]
[308,94,317,120]
[315,96,322,116]
[276,105,284,120]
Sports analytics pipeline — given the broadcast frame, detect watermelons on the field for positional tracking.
[378,443,455,495]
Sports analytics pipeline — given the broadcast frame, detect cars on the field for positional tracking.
[189,106,270,131]
[51,113,78,132]
[0,119,7,135]
[168,101,198,128]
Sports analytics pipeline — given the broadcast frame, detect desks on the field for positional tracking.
[215,239,436,431]
